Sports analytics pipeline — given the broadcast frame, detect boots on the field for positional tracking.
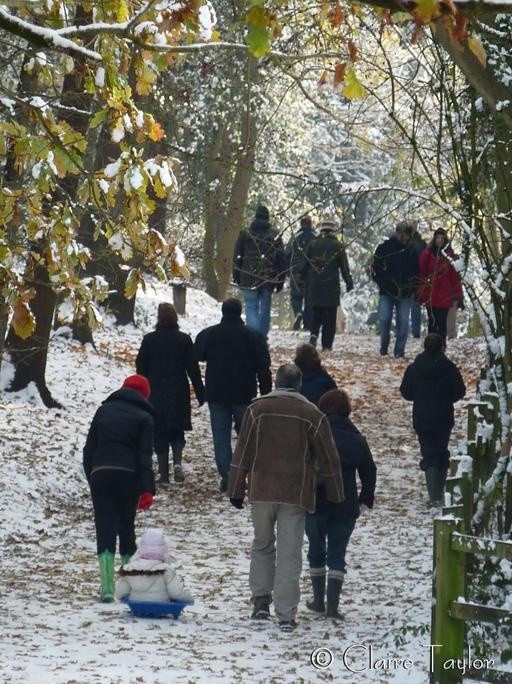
[155,463,168,483]
[306,568,326,615]
[326,570,347,620]
[97,550,115,601]
[174,461,184,482]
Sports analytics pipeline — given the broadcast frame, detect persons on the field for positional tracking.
[235,203,286,338]
[295,341,335,400]
[228,362,345,633]
[135,301,208,486]
[306,388,378,618]
[369,221,419,357]
[193,296,273,491]
[300,213,353,349]
[119,527,194,618]
[406,215,427,336]
[85,371,155,598]
[400,331,466,505]
[285,215,319,332]
[421,226,465,349]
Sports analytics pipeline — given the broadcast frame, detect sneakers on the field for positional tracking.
[220,477,230,491]
[395,353,404,359]
[321,347,333,355]
[280,620,298,632]
[310,334,317,348]
[251,604,270,619]
[293,312,302,330]
[380,346,388,356]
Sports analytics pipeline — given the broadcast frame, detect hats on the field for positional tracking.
[124,375,150,399]
[321,220,335,233]
[138,527,168,559]
[396,224,413,235]
[434,228,447,235]
[256,206,269,220]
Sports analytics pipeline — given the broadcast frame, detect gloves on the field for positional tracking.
[138,492,154,509]
[457,301,465,310]
[230,498,244,509]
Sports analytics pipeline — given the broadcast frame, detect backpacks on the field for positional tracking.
[240,228,279,286]
